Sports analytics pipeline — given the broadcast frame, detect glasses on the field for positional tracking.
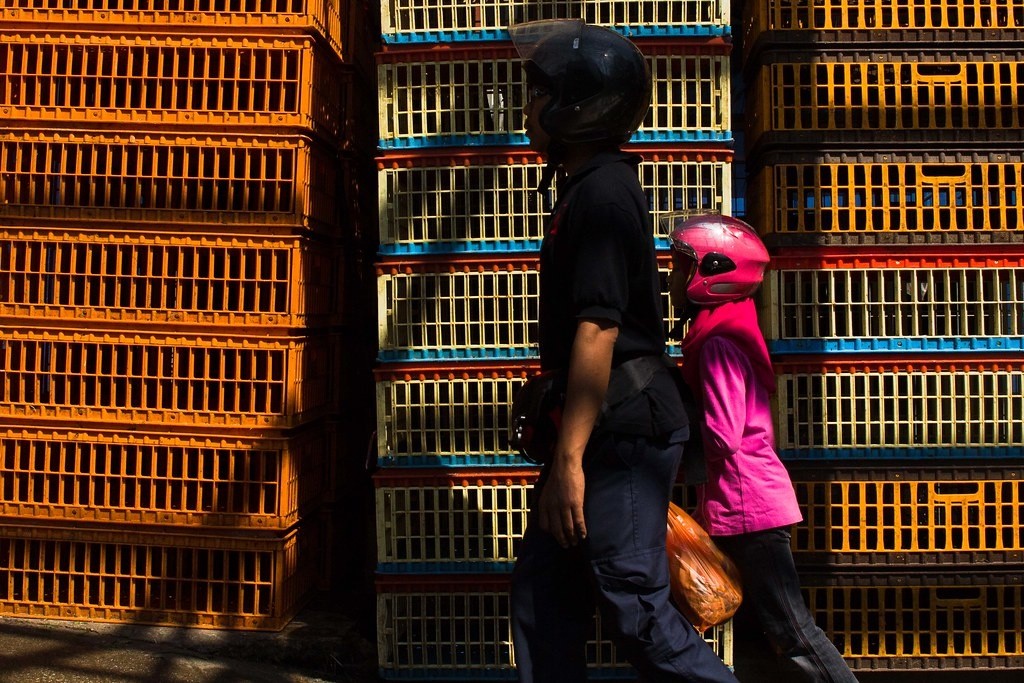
[527,81,551,99]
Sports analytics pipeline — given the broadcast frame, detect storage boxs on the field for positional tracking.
[1,0,1024,683]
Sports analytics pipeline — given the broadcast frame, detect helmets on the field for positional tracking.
[671,215,771,305]
[521,24,649,148]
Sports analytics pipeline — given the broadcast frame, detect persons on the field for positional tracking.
[659,208,859,682]
[507,16,741,683]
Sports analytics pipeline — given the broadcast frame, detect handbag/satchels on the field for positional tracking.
[664,501,743,635]
[512,372,563,462]
